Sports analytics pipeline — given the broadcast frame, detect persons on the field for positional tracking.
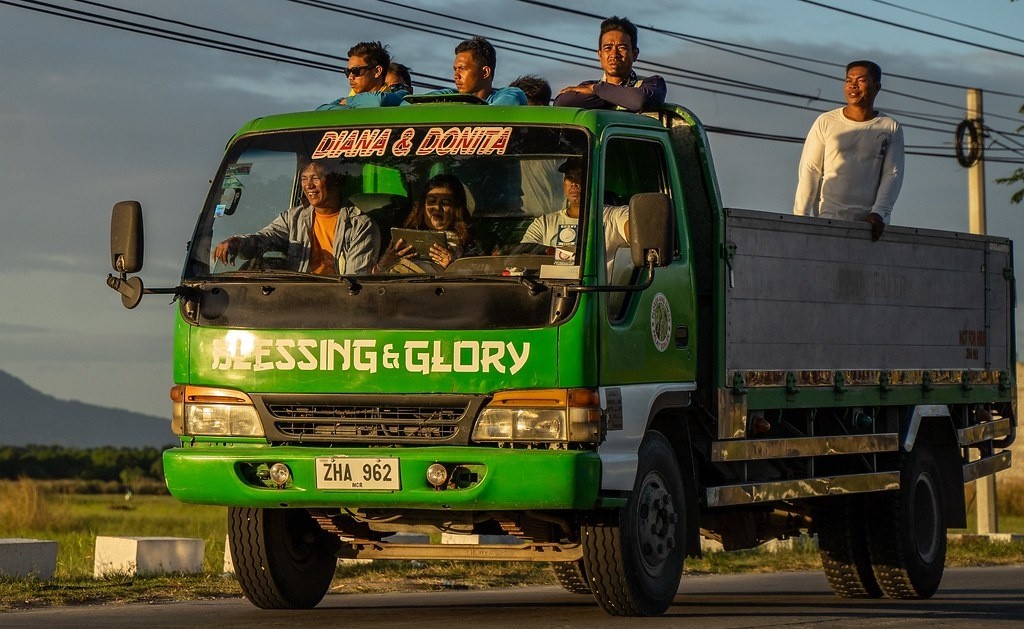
[791,60,906,244]
[369,174,490,278]
[397,35,527,106]
[212,153,380,276]
[507,156,659,291]
[316,40,413,112]
[509,74,551,106]
[553,16,668,114]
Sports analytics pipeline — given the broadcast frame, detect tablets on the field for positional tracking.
[392,227,448,260]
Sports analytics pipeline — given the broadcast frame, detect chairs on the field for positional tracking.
[349,194,412,265]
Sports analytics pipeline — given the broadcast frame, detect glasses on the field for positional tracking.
[344,65,378,78]
[388,83,410,92]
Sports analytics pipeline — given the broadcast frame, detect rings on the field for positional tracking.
[439,258,443,262]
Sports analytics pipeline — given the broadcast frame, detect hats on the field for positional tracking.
[558,155,584,172]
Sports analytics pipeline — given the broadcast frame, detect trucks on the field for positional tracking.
[107,94,1020,616]
